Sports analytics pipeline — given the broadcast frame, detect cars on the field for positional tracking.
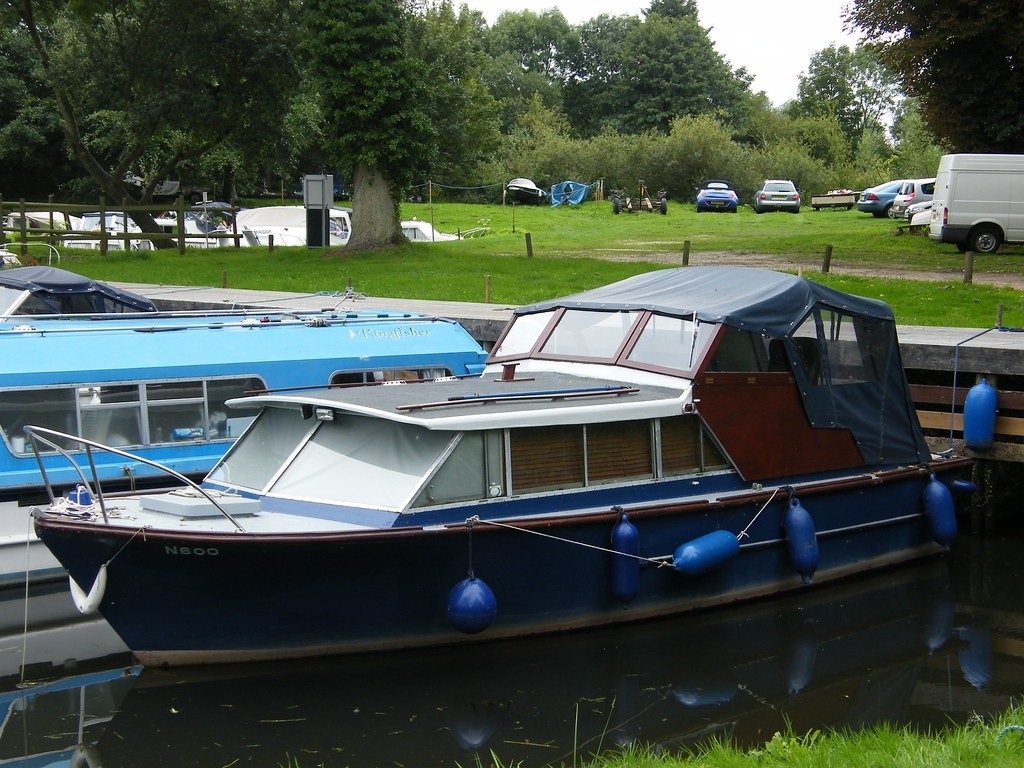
[856,180,910,219]
[903,200,933,223]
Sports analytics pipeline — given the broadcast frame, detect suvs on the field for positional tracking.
[892,178,937,219]
[753,178,800,215]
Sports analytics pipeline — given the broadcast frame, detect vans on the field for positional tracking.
[927,153,1024,255]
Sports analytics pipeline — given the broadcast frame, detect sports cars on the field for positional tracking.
[694,180,739,213]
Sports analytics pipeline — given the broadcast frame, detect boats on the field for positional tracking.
[0,266,158,318]
[124,176,180,200]
[0,584,127,682]
[1,556,969,767]
[0,307,492,590]
[22,265,986,672]
[0,201,463,250]
[506,177,548,204]
